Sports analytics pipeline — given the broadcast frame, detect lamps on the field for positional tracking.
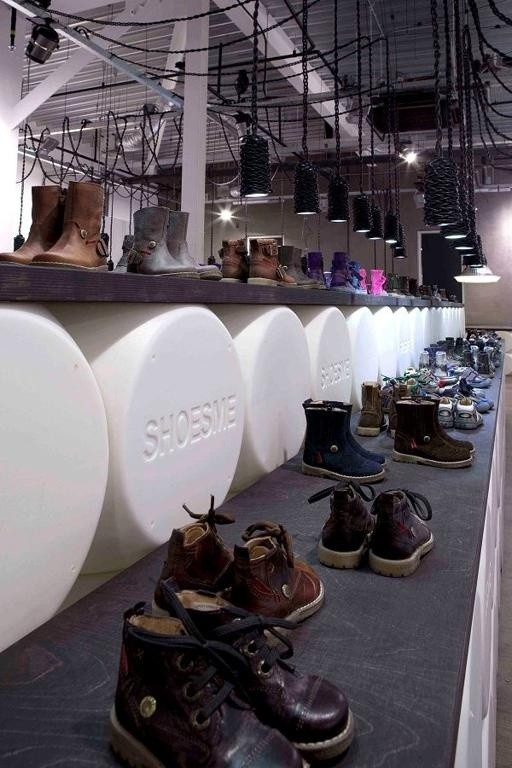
[422,2,489,270]
[236,1,274,204]
[291,1,321,218]
[325,1,352,227]
[25,16,58,65]
[348,1,413,259]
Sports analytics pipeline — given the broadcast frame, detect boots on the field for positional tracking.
[153,576,354,763]
[353,380,388,440]
[300,396,388,481]
[105,600,356,768]
[125,205,223,290]
[217,231,295,290]
[150,517,323,633]
[273,241,454,299]
[382,324,503,429]
[1,180,113,277]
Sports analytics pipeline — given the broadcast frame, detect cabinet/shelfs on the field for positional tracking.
[2,257,507,768]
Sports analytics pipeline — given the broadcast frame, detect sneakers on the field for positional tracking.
[316,482,374,573]
[368,489,436,580]
[389,393,476,470]
